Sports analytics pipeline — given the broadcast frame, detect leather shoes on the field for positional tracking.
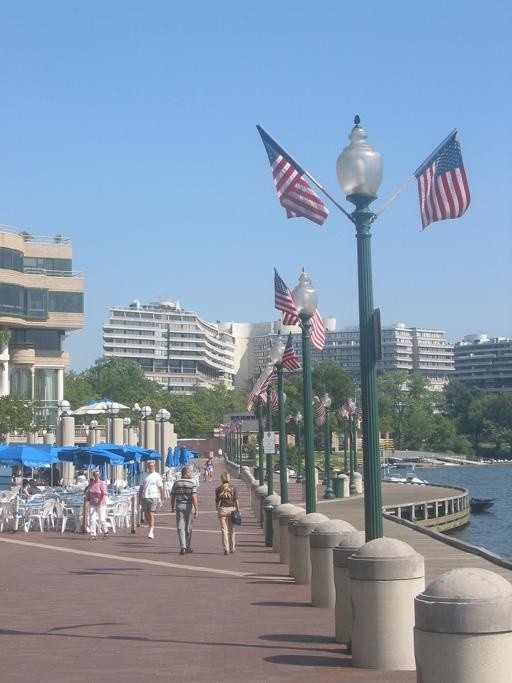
[181,547,194,555]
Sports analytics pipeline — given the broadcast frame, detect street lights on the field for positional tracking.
[218,417,243,478]
[290,266,319,512]
[336,111,385,654]
[53,398,173,486]
[253,362,360,509]
[268,327,288,505]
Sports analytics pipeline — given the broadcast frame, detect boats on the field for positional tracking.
[379,454,496,513]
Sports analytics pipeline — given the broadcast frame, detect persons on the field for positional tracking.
[170,467,199,554]
[215,473,240,555]
[204,456,214,481]
[138,460,164,538]
[86,470,109,541]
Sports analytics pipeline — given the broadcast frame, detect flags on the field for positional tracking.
[246,332,362,427]
[258,124,327,224]
[221,417,243,437]
[274,268,298,325]
[311,309,326,349]
[416,129,471,229]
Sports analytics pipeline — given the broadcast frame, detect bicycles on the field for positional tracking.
[201,464,209,483]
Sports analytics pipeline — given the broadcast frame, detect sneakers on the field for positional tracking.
[224,548,237,555]
[88,533,109,541]
[148,532,154,539]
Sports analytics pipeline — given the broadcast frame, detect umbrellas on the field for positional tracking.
[0,442,189,509]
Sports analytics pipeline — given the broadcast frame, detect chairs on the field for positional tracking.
[0,461,202,540]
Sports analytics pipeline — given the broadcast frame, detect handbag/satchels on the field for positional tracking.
[231,509,242,526]
